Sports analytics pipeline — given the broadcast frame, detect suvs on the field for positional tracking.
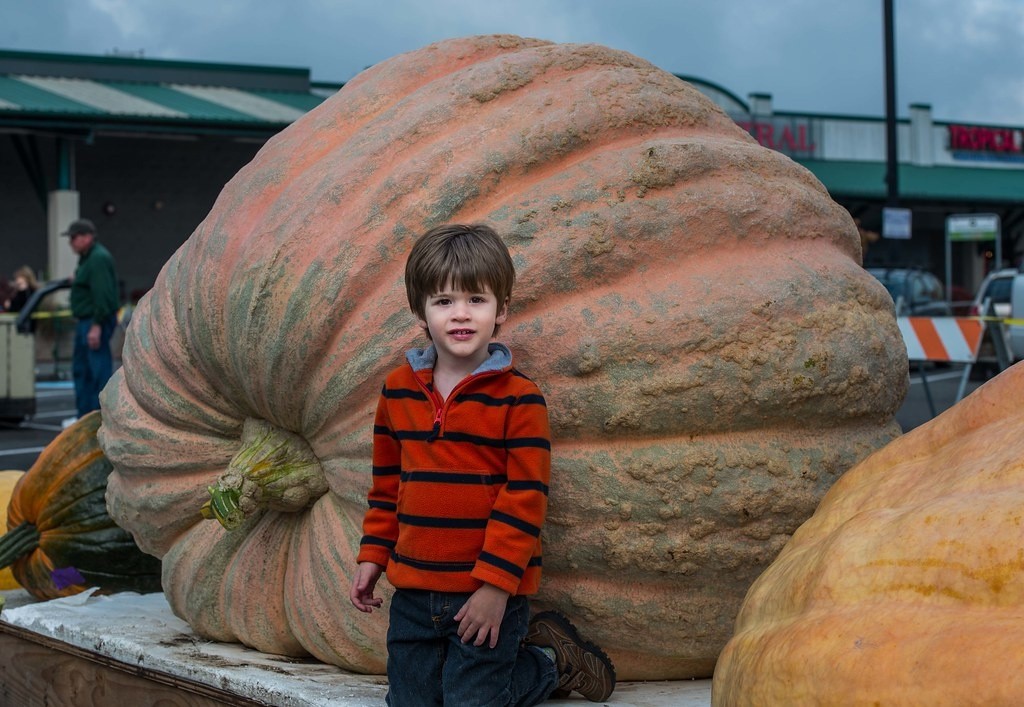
[866,266,953,371]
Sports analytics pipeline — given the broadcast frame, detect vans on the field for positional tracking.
[968,267,1024,380]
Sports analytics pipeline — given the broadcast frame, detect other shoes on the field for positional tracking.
[62,417,79,428]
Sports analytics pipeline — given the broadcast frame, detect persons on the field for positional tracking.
[63,218,118,428]
[5,267,43,312]
[351,224,615,707]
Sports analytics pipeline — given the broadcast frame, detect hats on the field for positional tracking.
[61,220,94,237]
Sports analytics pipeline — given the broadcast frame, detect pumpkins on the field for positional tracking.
[0,408,163,602]
[712,359,1024,707]
[97,29,907,682]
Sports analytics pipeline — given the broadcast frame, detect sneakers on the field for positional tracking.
[519,610,617,701]
